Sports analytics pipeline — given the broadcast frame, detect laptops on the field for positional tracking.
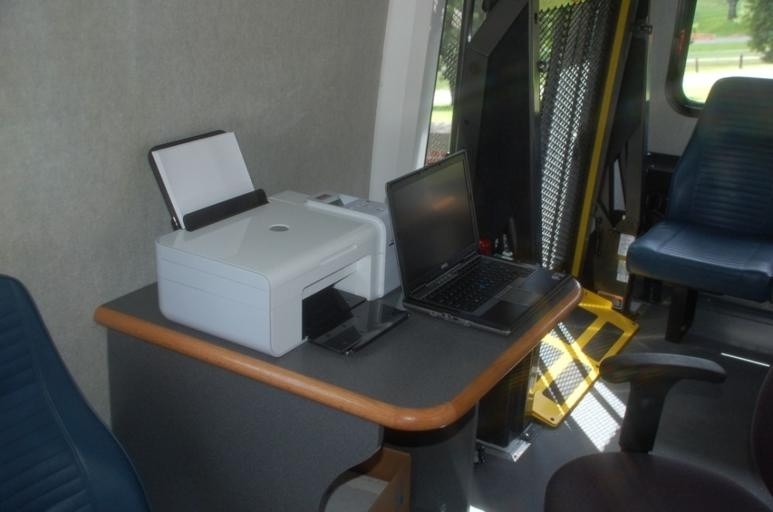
[385,148,574,336]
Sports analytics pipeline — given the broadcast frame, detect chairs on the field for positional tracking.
[626,75,771,340]
[542,351,771,511]
[0,277,149,512]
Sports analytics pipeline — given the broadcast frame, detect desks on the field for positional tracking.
[93,251,582,511]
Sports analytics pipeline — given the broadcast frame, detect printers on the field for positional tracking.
[147,130,409,358]
